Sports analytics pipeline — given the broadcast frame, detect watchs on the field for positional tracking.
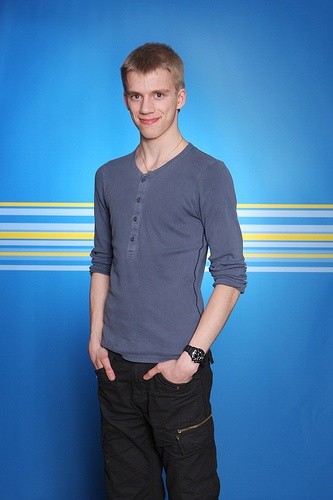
[184,345,205,363]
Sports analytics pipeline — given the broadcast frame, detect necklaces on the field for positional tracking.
[141,138,178,171]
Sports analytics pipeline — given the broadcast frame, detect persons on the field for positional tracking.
[88,43,249,500]
